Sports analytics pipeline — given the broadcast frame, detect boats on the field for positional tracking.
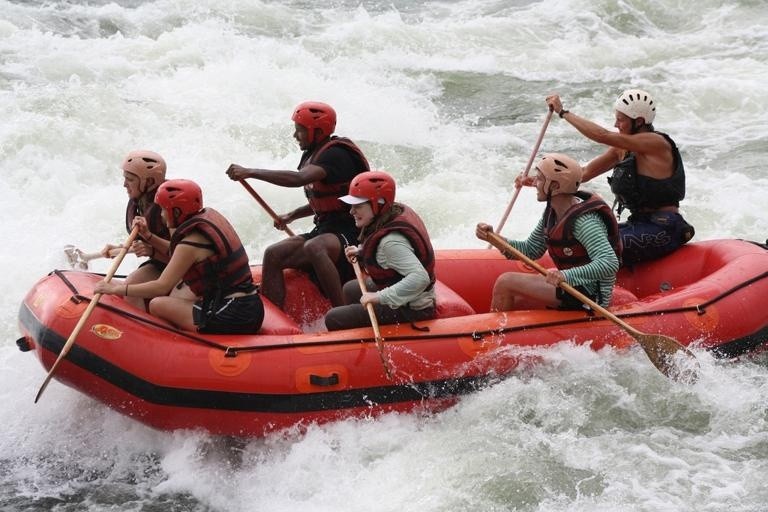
[13,232,767,443]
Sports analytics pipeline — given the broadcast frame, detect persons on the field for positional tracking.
[325,172,436,331]
[225,102,371,312]
[546,89,695,268]
[475,153,624,312]
[94,179,264,335]
[76,151,200,312]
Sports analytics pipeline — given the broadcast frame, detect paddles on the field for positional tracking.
[350,256,392,380]
[485,231,700,386]
[35,224,140,403]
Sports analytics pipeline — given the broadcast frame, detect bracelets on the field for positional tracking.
[145,233,152,241]
[126,284,128,296]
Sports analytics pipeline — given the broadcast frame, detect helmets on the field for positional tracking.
[537,153,582,196]
[349,171,396,216]
[292,103,336,144]
[118,149,166,193]
[614,89,657,124]
[154,180,202,223]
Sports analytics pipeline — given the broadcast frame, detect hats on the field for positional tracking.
[338,194,388,205]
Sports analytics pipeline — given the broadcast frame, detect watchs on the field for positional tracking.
[559,109,569,118]
